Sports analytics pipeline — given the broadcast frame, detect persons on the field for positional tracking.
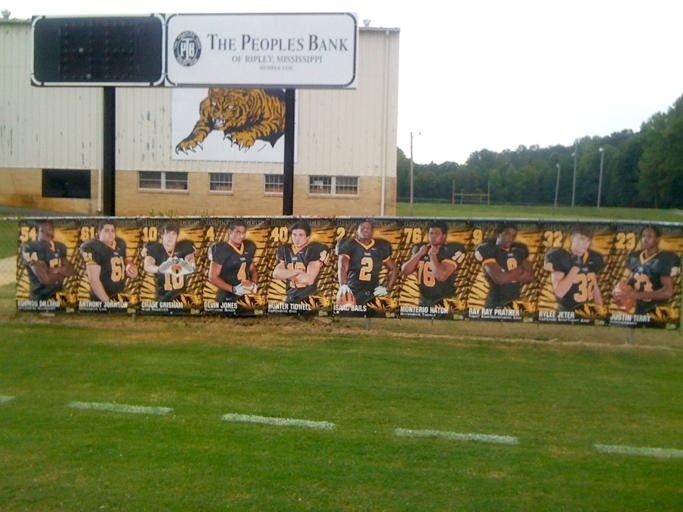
[270,220,330,309]
[399,223,467,313]
[19,221,79,307]
[473,223,537,309]
[138,220,200,308]
[73,222,138,306]
[607,226,682,317]
[541,224,604,317]
[204,220,260,307]
[332,219,396,307]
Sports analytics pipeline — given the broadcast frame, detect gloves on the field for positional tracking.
[373,286,388,297]
[157,256,179,277]
[232,283,252,296]
[177,257,195,277]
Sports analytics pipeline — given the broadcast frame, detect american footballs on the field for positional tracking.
[613,282,636,313]
[124,260,135,277]
[337,292,357,306]
[241,280,253,288]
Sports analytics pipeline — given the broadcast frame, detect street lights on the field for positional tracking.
[551,146,605,209]
